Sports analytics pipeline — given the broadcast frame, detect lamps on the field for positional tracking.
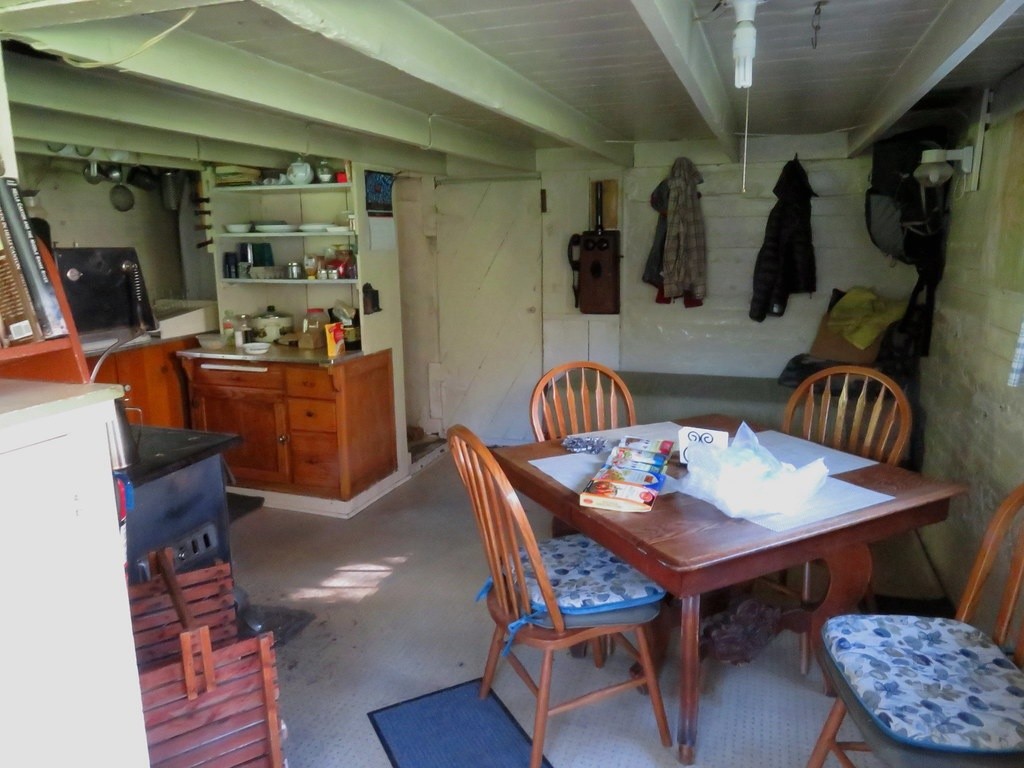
[914,145,974,187]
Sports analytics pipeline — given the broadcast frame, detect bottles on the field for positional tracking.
[287,262,303,279]
[304,243,357,279]
[298,308,331,349]
[317,159,336,182]
[223,310,236,345]
[223,252,237,278]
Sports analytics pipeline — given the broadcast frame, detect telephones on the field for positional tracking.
[567,233,581,271]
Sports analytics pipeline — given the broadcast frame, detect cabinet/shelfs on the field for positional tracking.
[175,162,409,515]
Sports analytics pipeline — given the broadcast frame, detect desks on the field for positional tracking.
[490,414,964,764]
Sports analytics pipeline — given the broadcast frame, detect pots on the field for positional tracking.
[84,164,156,212]
[250,304,295,343]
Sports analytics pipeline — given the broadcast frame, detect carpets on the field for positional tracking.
[367,678,553,768]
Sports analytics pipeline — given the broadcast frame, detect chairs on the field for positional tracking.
[806,485,1024,768]
[529,361,636,656]
[749,365,912,675]
[447,424,673,768]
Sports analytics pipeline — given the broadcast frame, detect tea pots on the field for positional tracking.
[286,157,315,185]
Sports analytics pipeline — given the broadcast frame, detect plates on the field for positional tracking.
[242,343,271,355]
[326,225,348,232]
[255,224,300,232]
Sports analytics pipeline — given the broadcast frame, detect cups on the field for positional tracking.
[238,263,251,279]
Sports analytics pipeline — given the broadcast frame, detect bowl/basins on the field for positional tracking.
[224,223,252,233]
[195,333,231,350]
[300,224,337,232]
